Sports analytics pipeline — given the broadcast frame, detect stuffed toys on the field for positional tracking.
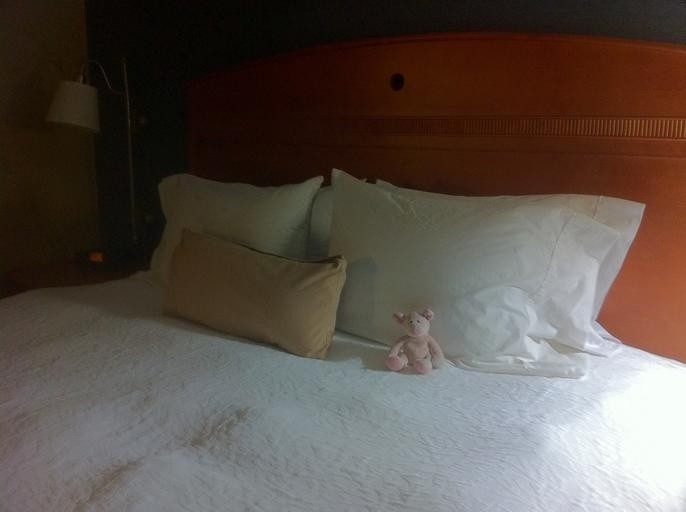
[386,308,444,373]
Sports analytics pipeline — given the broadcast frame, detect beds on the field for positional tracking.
[0,31,686,512]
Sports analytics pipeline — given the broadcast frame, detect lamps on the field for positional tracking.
[47,53,143,247]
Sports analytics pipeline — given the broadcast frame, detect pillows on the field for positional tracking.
[165,227,347,359]
[148,166,646,362]
[149,173,324,290]
[313,168,645,379]
